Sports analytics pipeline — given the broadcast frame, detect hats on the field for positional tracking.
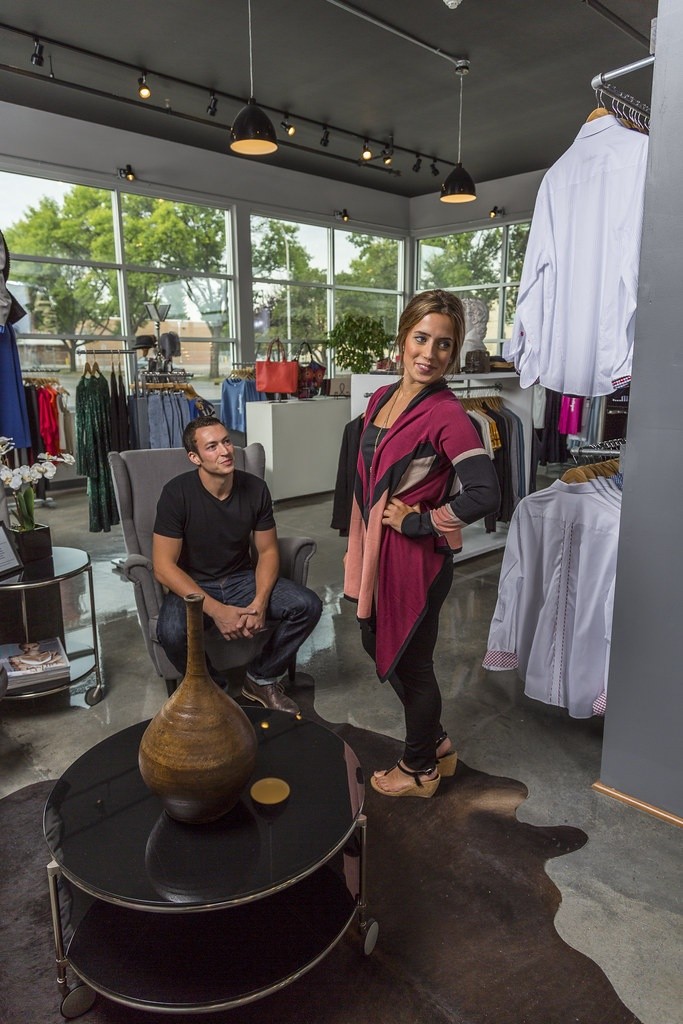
[131,334,154,349]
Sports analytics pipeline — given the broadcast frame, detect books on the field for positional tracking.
[0,637,70,690]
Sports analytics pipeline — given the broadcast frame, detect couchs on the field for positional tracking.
[108,443,317,687]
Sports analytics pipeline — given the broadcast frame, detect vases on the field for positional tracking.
[8,523,53,561]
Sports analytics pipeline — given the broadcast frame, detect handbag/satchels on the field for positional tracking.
[287,341,326,398]
[256,338,298,393]
[465,350,490,374]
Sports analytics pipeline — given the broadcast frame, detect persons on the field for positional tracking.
[151,416,322,715]
[7,642,63,673]
[343,289,501,799]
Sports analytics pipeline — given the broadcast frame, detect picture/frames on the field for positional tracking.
[0,520,25,577]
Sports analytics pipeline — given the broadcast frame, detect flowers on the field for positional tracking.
[0,462,61,530]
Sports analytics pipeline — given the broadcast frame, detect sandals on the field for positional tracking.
[370,731,457,796]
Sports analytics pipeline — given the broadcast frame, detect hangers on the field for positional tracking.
[581,73,651,144]
[135,370,196,396]
[77,349,124,382]
[530,436,626,503]
[224,359,263,385]
[19,366,64,397]
[449,382,516,419]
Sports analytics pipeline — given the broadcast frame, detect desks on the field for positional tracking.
[42,701,376,1018]
[0,544,105,706]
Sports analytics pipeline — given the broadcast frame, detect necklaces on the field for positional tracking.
[369,388,399,474]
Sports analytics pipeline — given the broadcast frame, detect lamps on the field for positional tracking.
[382,144,393,165]
[412,154,423,173]
[342,208,349,222]
[440,59,476,205]
[229,0,277,154]
[429,157,440,178]
[491,207,505,219]
[137,71,151,98]
[118,164,134,182]
[363,138,372,159]
[319,126,331,146]
[31,36,45,66]
[280,115,296,135]
[206,89,218,116]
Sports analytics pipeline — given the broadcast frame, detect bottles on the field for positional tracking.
[143,809,259,906]
[138,594,258,826]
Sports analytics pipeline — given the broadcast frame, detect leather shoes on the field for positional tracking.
[243,675,301,715]
